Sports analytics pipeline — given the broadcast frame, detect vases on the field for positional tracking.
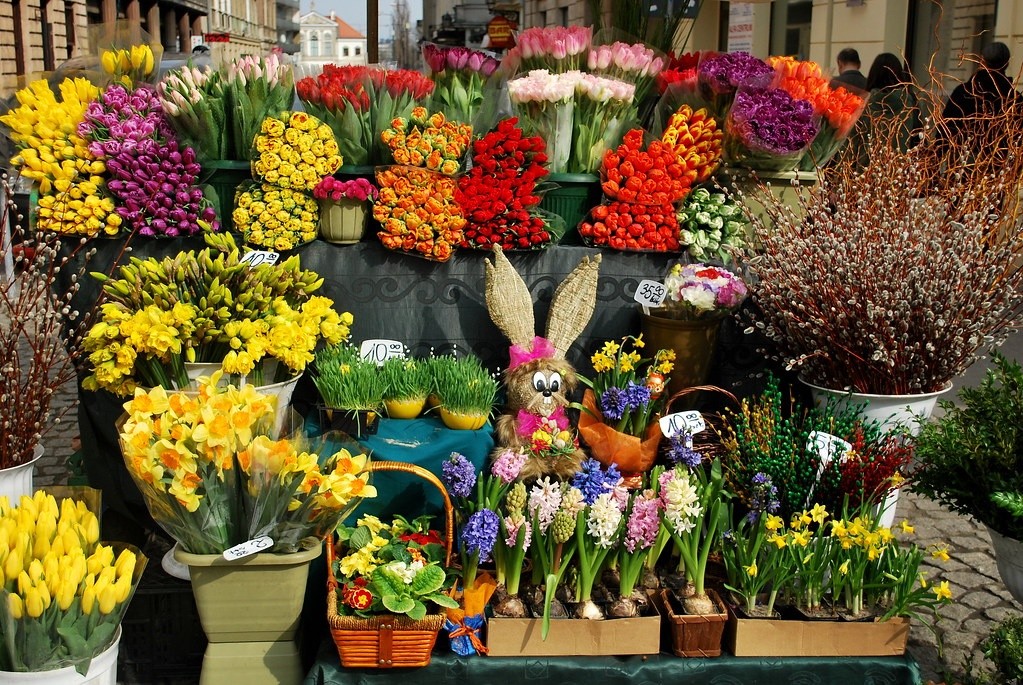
[0,161,951,685]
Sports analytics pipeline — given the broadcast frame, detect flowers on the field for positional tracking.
[0,25,1023,625]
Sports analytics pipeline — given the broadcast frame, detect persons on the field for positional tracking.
[928,42,1023,255]
[854,52,928,198]
[822,48,868,179]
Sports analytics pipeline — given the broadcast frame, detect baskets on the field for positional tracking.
[326,459,454,668]
